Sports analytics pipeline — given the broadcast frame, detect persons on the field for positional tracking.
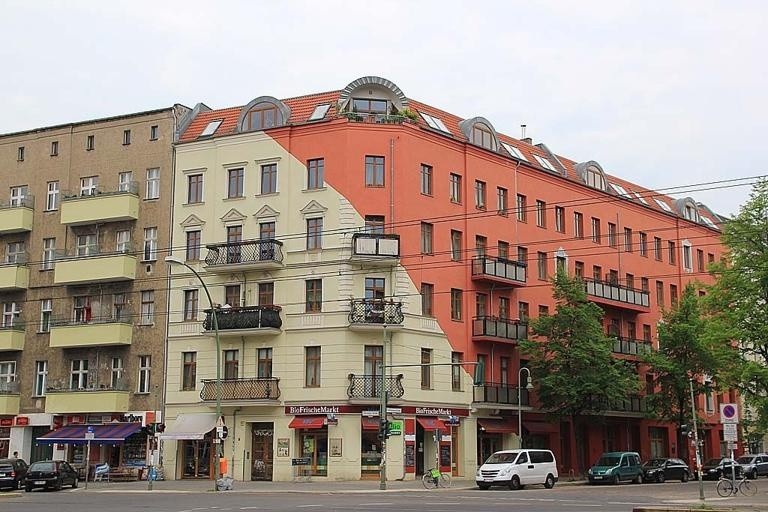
[13,451,19,458]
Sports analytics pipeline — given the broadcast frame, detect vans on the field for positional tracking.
[584,451,644,487]
[474,446,559,492]
[0,457,28,492]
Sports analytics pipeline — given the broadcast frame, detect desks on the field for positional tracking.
[74,467,84,478]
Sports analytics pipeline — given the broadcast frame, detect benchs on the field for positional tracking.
[95,472,130,483]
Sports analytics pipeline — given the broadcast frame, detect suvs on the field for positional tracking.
[733,452,767,480]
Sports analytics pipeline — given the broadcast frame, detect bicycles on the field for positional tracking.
[714,473,757,499]
[420,467,451,491]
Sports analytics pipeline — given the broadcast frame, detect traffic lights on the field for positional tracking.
[155,422,165,434]
[378,419,390,440]
[145,422,155,437]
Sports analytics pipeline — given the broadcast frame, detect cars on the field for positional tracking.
[692,458,741,482]
[639,457,690,485]
[22,460,80,494]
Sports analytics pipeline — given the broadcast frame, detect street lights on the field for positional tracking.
[516,365,534,449]
[162,254,225,494]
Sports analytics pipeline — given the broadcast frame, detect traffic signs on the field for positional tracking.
[360,410,379,417]
[385,407,401,414]
